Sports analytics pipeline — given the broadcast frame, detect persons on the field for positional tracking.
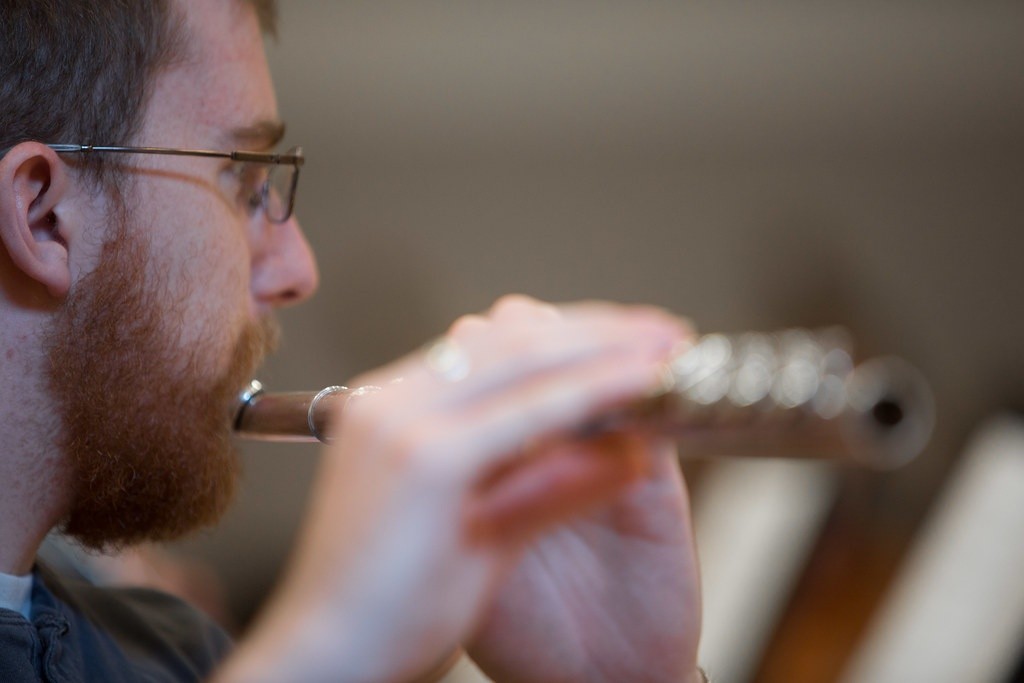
[0,0,705,683]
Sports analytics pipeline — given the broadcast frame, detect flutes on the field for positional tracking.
[230,329,937,473]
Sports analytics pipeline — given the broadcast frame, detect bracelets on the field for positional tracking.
[696,666,711,683]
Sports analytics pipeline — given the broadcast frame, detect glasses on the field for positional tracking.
[0,143,306,226]
